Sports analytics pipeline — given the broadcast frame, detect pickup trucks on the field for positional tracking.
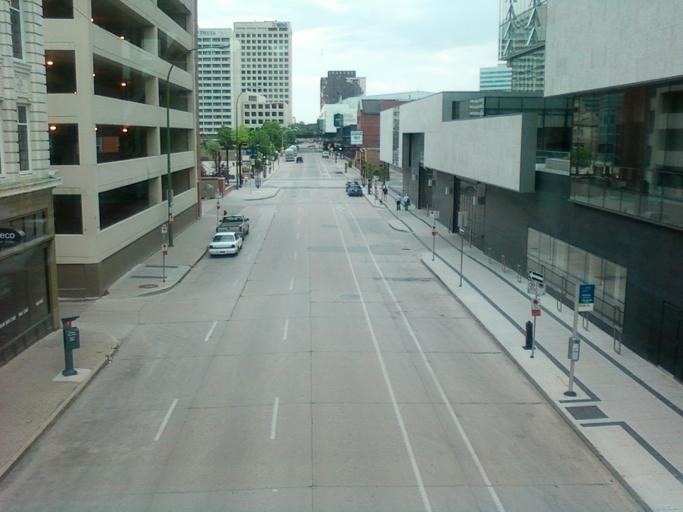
[215,216,250,242]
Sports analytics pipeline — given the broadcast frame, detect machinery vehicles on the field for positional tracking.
[283,145,304,164]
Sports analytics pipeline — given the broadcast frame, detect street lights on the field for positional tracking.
[234,87,268,188]
[163,43,230,248]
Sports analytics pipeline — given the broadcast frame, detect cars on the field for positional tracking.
[344,180,362,198]
[208,231,243,257]
[322,151,329,158]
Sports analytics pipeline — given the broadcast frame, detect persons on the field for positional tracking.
[382,185,388,201]
[394,193,400,211]
[402,192,409,211]
[367,178,371,193]
